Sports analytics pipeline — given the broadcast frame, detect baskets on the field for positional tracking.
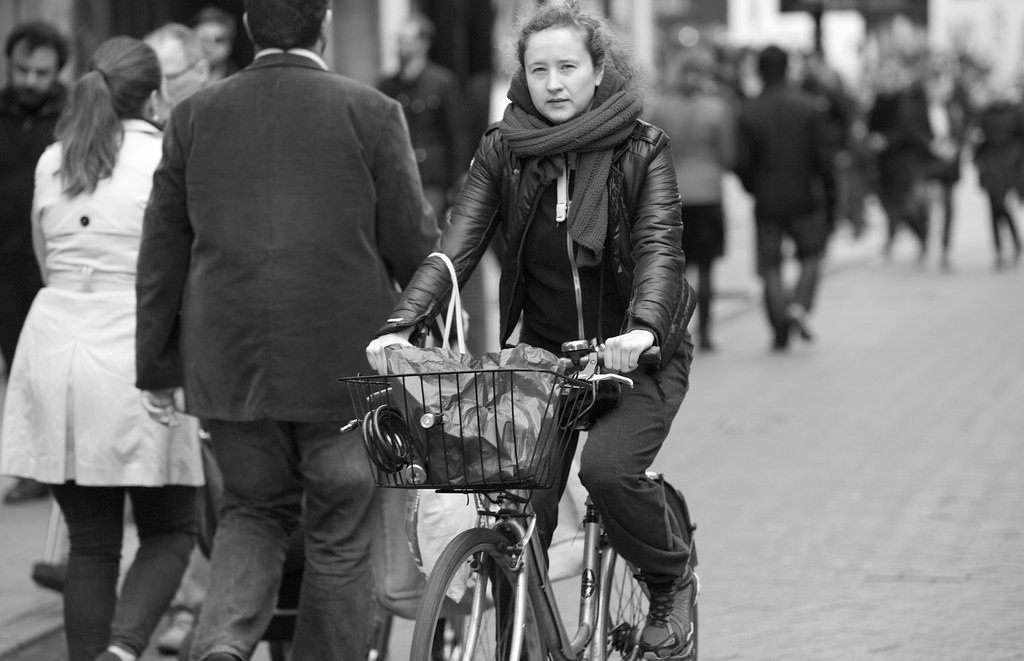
[338,368,590,493]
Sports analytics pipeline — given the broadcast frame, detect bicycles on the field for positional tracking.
[337,329,700,661]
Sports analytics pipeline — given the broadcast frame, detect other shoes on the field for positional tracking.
[4,479,50,501]
[776,328,789,347]
[157,609,196,655]
[32,562,65,592]
[786,303,813,341]
[95,649,139,661]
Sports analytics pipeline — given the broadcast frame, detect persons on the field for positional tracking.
[0,1,1024,660]
[734,45,841,354]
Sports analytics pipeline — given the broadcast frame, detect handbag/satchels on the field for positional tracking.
[404,488,478,602]
[476,466,585,604]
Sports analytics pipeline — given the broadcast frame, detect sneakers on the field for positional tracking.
[632,573,702,660]
